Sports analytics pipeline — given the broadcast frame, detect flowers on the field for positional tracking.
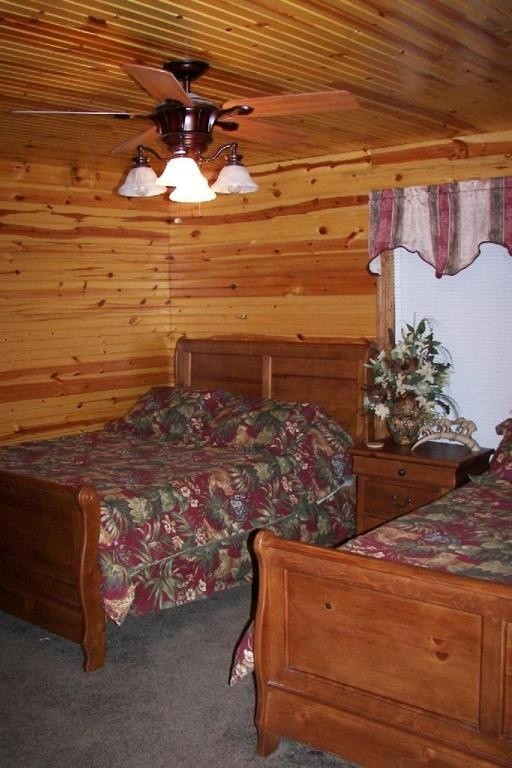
[359,311,460,422]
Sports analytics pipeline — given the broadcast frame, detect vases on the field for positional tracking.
[387,390,419,448]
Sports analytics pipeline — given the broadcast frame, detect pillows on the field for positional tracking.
[466,418,511,501]
[104,385,358,458]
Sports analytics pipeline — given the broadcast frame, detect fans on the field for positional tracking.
[10,59,363,159]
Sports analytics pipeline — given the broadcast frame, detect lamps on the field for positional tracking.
[118,142,260,204]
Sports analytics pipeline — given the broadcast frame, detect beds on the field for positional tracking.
[0,336,380,673]
[253,470,512,768]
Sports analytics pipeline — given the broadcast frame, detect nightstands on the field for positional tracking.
[347,436,495,538]
